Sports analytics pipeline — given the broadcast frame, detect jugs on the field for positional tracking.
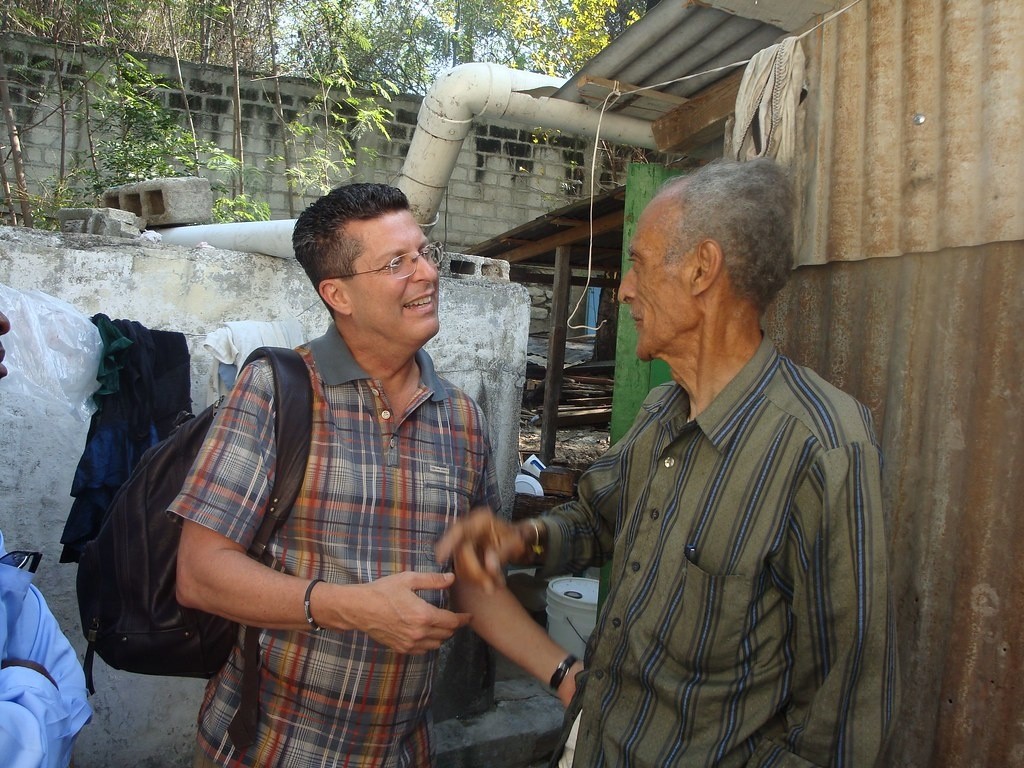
[521,453,546,479]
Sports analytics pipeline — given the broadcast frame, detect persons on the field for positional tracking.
[0,311,94,768]
[165,184,586,768]
[436,156,901,768]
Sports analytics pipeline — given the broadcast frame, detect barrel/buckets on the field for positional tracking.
[545,576,600,668]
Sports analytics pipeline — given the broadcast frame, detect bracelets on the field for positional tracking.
[548,655,576,692]
[304,579,326,630]
[530,519,544,554]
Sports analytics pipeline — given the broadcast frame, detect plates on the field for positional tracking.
[513,474,544,496]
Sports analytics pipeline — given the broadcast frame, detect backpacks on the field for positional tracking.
[76,347,314,693]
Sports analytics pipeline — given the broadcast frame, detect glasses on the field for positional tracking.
[323,241,444,280]
